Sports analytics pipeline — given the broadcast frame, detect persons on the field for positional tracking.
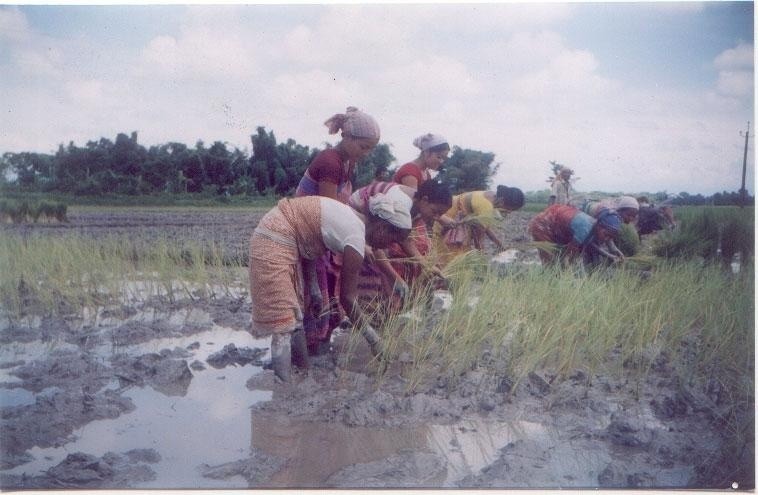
[247,104,454,380]
[431,184,523,256]
[527,164,672,269]
